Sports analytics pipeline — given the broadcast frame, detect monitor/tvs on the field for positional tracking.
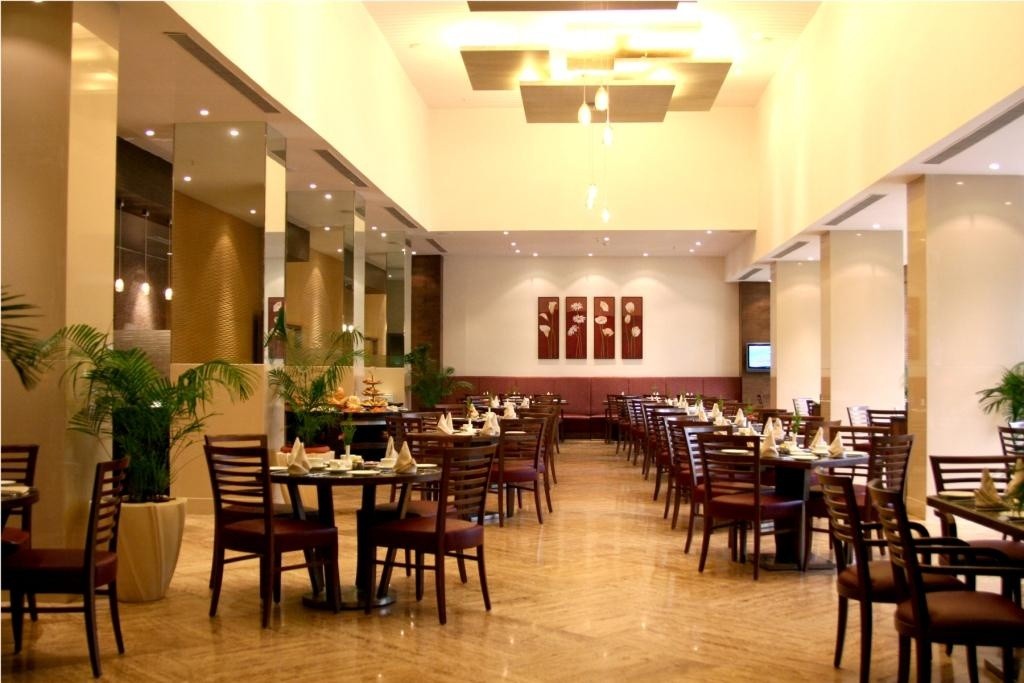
[746,343,771,373]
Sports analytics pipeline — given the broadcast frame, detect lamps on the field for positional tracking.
[455,19,734,155]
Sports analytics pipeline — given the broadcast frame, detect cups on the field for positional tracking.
[340,455,355,460]
[381,458,396,466]
[329,460,345,468]
[463,424,472,431]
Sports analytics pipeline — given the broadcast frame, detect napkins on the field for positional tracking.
[762,416,773,435]
[485,393,500,404]
[284,434,312,477]
[761,431,779,458]
[694,408,708,421]
[378,433,398,467]
[733,406,746,425]
[519,396,529,409]
[390,439,419,477]
[710,402,720,418]
[677,395,683,407]
[773,416,784,435]
[829,429,846,457]
[479,411,501,439]
[969,464,1011,514]
[502,402,516,420]
[808,427,827,452]
[435,410,454,435]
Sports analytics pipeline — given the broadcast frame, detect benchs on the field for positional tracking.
[445,374,747,440]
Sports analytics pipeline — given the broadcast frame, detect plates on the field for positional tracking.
[417,464,437,467]
[377,465,394,469]
[311,465,380,475]
[938,491,975,497]
[999,511,1024,520]
[1,480,16,484]
[721,449,867,461]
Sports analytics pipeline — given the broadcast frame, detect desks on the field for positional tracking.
[720,445,870,572]
[286,402,413,455]
[2,485,41,551]
[923,491,1024,534]
[252,460,443,611]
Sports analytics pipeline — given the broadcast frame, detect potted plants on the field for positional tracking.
[35,321,264,607]
[261,304,375,509]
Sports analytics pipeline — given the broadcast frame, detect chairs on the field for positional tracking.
[1,442,44,624]
[202,389,571,632]
[599,386,1024,683]
[24,456,132,680]
